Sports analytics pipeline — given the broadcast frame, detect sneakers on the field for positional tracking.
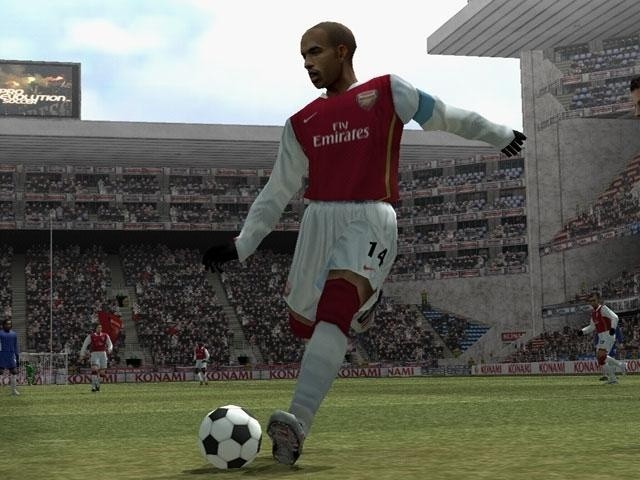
[266,410,307,466]
[608,379,618,385]
[349,288,383,334]
[12,390,20,395]
[620,361,626,373]
[92,382,100,392]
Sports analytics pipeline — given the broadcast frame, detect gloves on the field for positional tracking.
[501,130,528,158]
[577,330,584,337]
[201,239,238,274]
[609,328,616,336]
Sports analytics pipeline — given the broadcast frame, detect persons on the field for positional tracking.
[77,323,114,393]
[512,159,640,362]
[192,339,212,385]
[629,74,639,121]
[573,291,631,389]
[0,317,22,395]
[1,163,525,371]
[189,18,531,471]
